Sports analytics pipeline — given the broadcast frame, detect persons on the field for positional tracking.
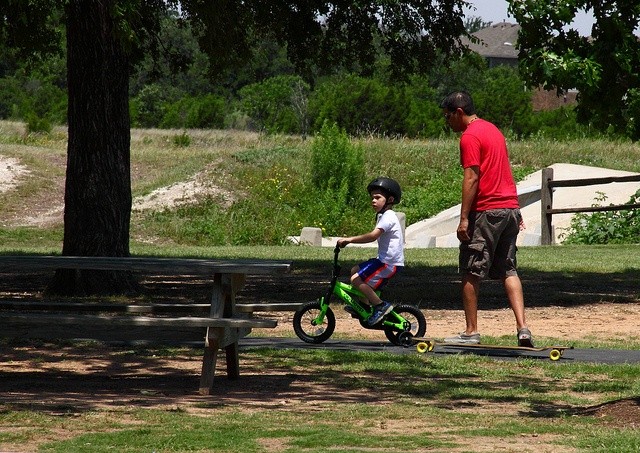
[438,90,535,348]
[337,177,405,327]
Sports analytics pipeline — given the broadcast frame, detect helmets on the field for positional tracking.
[367,177,401,204]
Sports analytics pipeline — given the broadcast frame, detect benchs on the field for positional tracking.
[0,256,303,396]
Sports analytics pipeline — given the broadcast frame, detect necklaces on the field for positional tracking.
[467,117,478,126]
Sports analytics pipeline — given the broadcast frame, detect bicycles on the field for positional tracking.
[292,239,427,347]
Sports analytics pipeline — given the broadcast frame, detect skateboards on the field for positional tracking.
[411,337,574,360]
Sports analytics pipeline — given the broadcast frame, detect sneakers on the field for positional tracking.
[368,301,394,326]
[444,331,481,345]
[345,300,371,315]
[518,328,534,348]
